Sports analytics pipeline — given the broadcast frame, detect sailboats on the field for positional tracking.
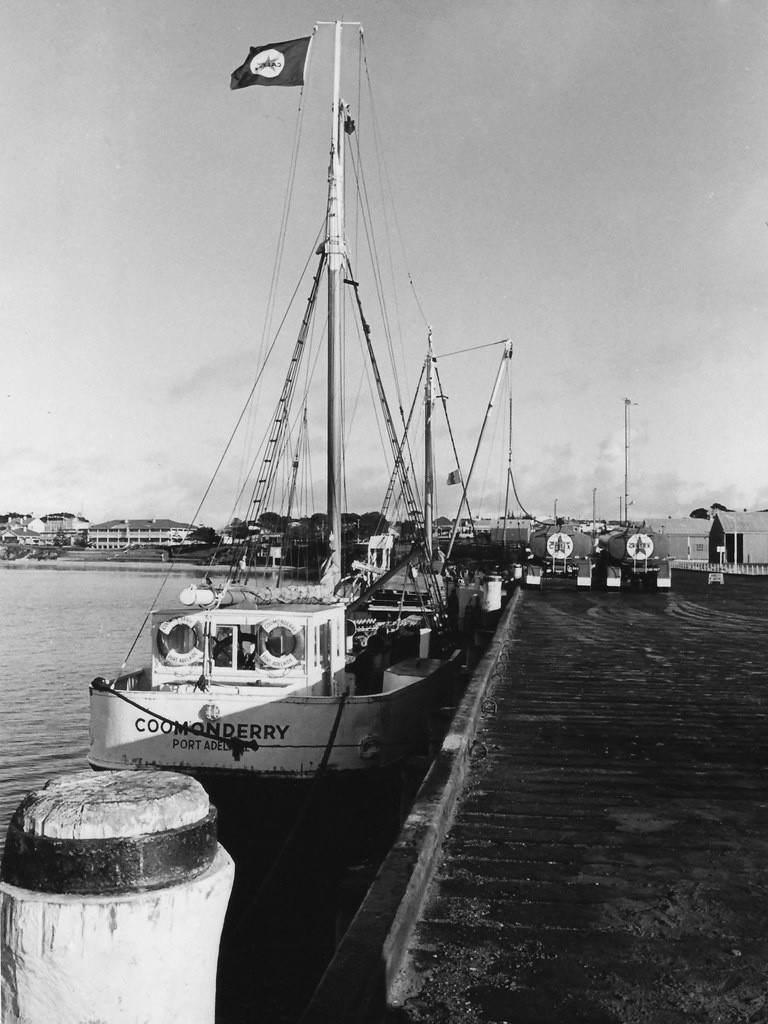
[339,324,522,640]
[86,20,465,781]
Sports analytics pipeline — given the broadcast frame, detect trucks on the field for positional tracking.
[525,518,674,592]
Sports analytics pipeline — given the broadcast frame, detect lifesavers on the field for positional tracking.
[256,615,306,670]
[156,616,205,665]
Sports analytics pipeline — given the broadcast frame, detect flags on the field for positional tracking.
[229,36,311,90]
